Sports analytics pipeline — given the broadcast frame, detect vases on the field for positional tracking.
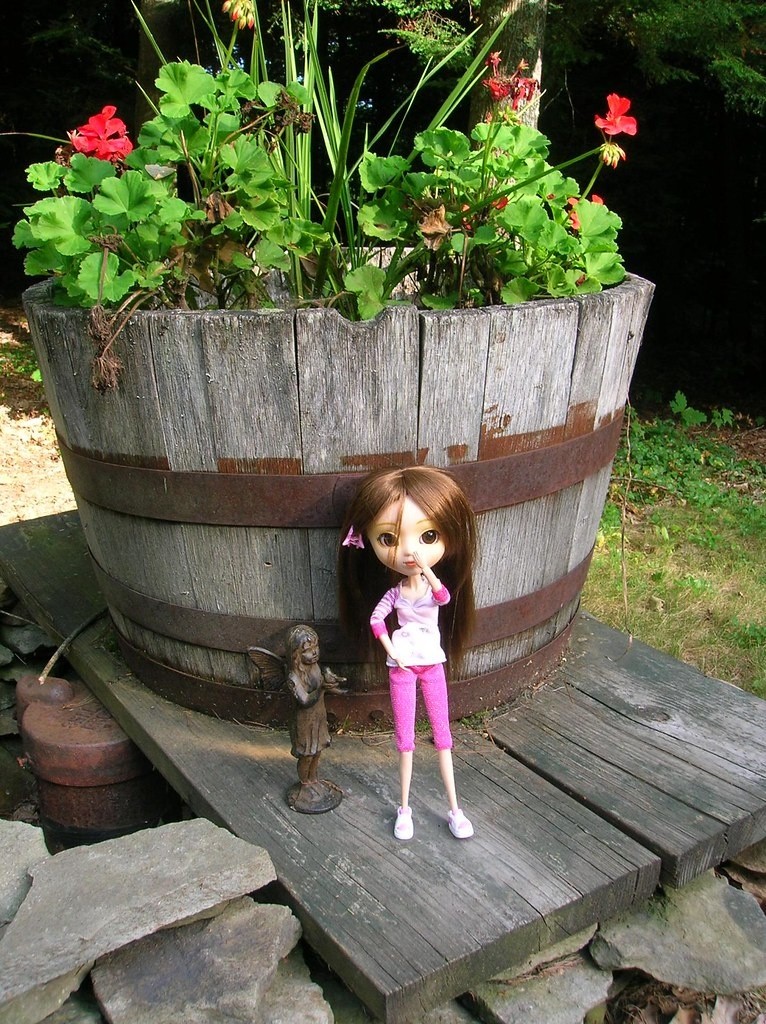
[21,247,656,734]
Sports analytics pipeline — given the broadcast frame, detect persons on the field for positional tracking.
[336,466,482,841]
[277,624,348,814]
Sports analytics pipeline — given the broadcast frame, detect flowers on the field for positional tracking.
[0,1,639,310]
